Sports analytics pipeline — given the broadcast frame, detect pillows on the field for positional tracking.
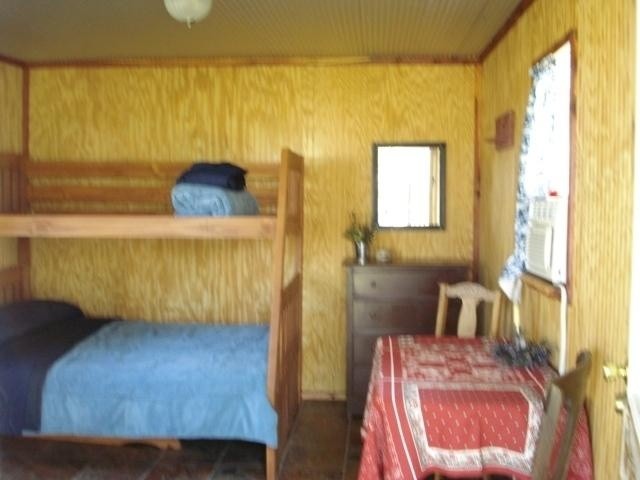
[1,300,86,347]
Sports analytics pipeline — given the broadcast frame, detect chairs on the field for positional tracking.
[433,278,503,340]
[526,348,594,480]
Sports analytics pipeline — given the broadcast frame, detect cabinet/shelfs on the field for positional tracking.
[338,256,472,425]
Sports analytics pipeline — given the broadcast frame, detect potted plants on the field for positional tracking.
[340,211,378,266]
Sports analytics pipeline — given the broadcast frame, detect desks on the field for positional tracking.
[359,334,595,480]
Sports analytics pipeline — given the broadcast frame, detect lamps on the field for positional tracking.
[162,0,214,30]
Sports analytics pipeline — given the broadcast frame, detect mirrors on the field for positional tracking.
[372,141,448,230]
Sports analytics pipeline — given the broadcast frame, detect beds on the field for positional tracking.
[1,146,308,480]
